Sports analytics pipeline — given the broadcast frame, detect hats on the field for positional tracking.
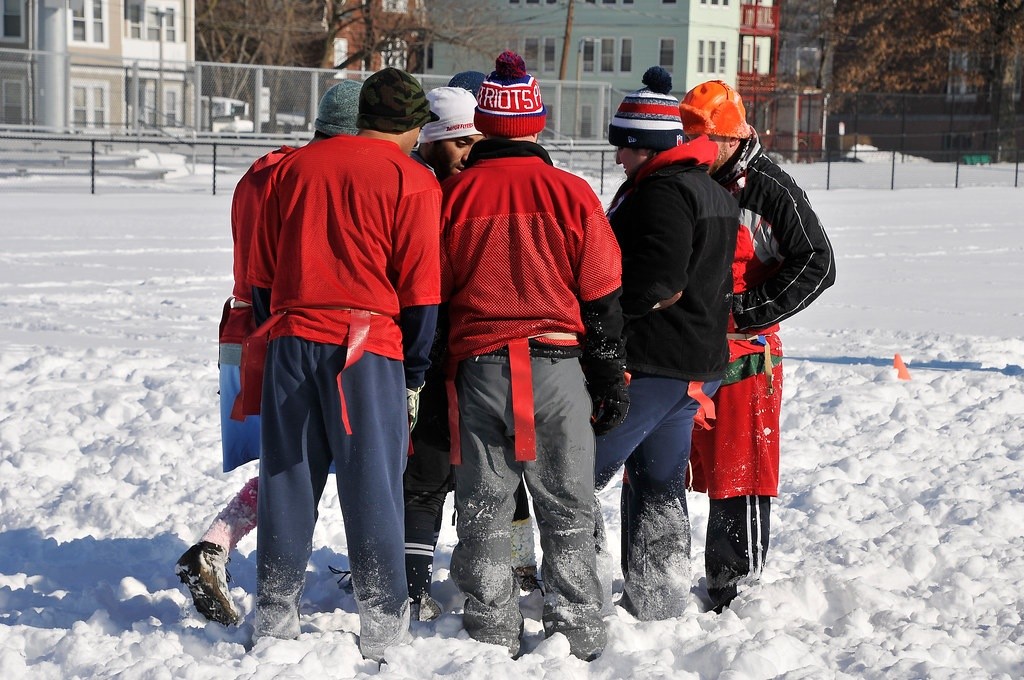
[356,67,440,130]
[608,65,684,149]
[313,80,362,136]
[680,80,751,138]
[416,86,482,143]
[448,71,486,99]
[473,50,546,138]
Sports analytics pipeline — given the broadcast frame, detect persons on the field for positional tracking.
[246,68,444,662]
[593,65,739,621]
[677,79,835,613]
[441,53,632,664]
[413,72,540,624]
[175,80,365,627]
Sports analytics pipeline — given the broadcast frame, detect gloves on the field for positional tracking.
[589,357,631,437]
[405,380,426,433]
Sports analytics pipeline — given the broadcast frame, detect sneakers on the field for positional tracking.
[511,565,545,599]
[175,541,243,627]
[327,564,354,595]
